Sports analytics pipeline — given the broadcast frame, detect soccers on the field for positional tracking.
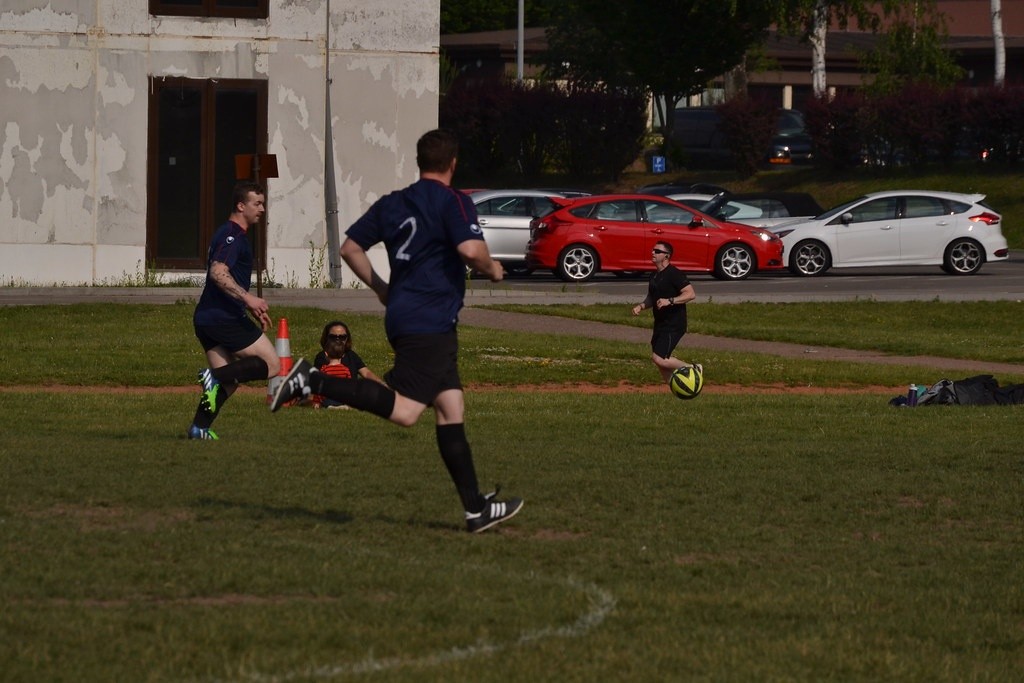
[668,366,703,400]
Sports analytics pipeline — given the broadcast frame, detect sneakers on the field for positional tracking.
[189,424,219,440]
[464,484,524,532]
[271,358,320,412]
[196,369,220,413]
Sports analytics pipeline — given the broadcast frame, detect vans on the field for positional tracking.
[662,106,816,168]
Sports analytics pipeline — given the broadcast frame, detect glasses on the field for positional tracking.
[652,248,670,254]
[326,333,349,341]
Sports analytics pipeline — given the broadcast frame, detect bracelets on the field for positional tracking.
[381,382,386,386]
[639,304,642,310]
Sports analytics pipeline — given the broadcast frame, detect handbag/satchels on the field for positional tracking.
[889,394,907,405]
[954,374,999,405]
[313,365,352,404]
[916,385,928,400]
[917,378,956,405]
[995,383,1024,404]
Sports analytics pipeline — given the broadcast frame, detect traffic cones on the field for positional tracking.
[263,317,298,408]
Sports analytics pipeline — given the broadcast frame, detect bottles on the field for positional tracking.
[908,384,918,407]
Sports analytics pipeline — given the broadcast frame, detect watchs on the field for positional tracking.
[668,297,674,305]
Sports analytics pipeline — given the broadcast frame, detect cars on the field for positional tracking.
[524,194,786,283]
[764,188,1010,277]
[457,180,827,279]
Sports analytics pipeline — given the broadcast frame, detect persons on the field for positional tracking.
[271,129,524,534]
[632,241,702,385]
[189,180,280,438]
[281,320,390,410]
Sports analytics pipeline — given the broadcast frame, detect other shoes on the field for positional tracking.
[692,362,704,373]
